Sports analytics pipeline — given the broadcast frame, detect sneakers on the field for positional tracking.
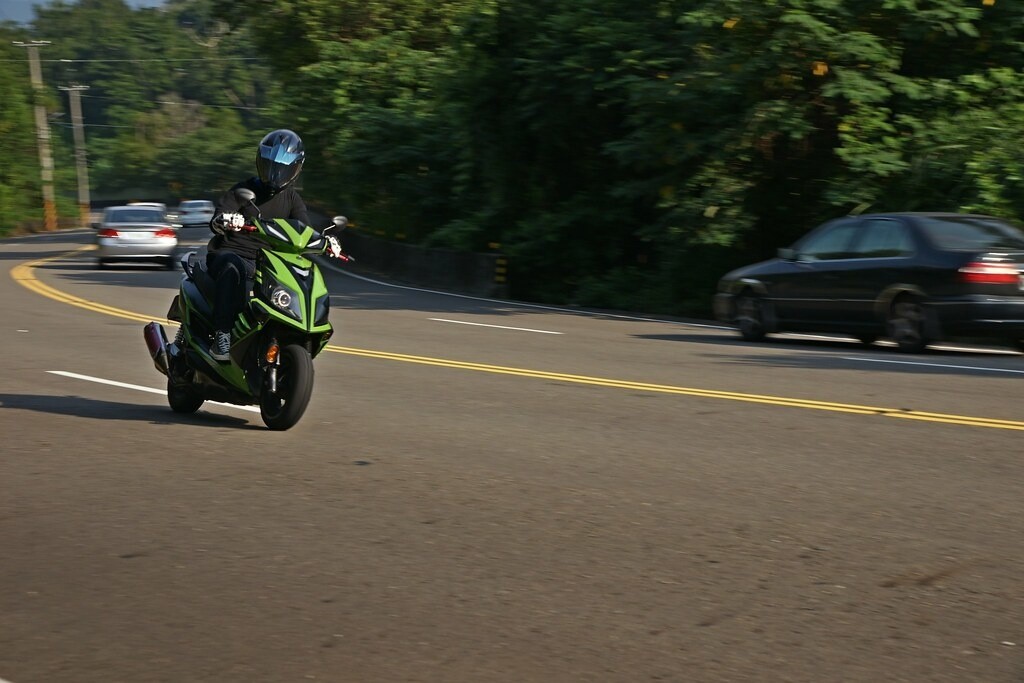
[208,329,230,361]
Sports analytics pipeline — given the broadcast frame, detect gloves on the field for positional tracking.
[223,212,245,231]
[325,236,341,258]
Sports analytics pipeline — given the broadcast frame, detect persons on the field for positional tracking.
[207,130,341,361]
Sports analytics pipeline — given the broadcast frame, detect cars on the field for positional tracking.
[714,211,1024,354]
[180,199,217,226]
[90,203,183,270]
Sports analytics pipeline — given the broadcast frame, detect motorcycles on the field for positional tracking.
[144,188,355,429]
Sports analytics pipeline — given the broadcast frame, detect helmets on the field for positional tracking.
[256,129,305,195]
